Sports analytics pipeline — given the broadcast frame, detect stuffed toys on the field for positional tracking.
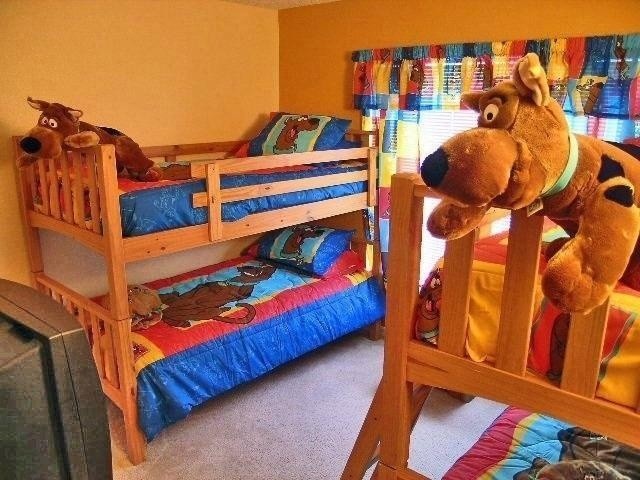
[14,96,164,183]
[418,51,639,314]
[99,282,168,328]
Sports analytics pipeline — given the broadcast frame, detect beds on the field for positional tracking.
[340,170,639,480]
[10,111,381,467]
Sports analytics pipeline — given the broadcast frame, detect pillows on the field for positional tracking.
[233,112,352,170]
[246,224,356,277]
[321,248,367,279]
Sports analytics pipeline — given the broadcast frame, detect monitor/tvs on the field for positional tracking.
[0,278,113,480]
